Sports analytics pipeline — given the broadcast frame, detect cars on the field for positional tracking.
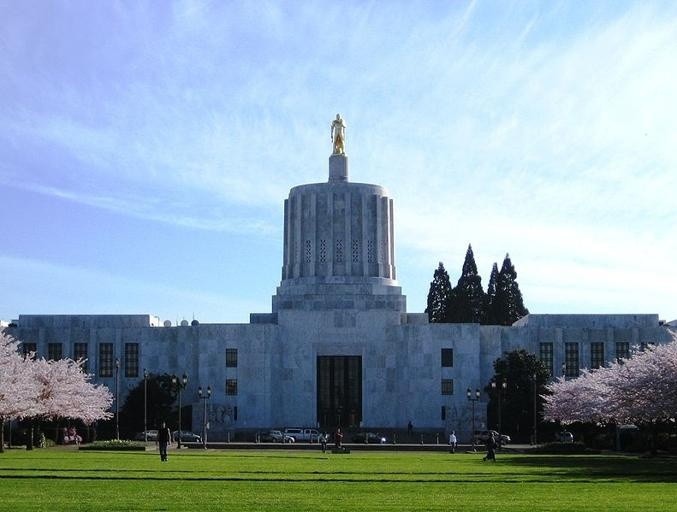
[560,432,573,443]
[170,430,202,442]
[352,432,386,443]
[136,430,159,441]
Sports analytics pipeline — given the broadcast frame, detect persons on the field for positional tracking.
[155,421,172,461]
[481,432,496,462]
[333,427,343,449]
[318,430,327,453]
[330,112,346,156]
[407,420,413,437]
[447,430,457,453]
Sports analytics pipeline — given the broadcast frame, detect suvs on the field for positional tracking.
[266,430,295,443]
[471,430,511,445]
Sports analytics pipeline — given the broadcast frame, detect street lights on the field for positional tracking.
[115,358,120,440]
[528,372,537,445]
[466,387,480,453]
[198,385,211,449]
[143,368,148,442]
[490,377,508,451]
[561,362,566,449]
[171,373,187,449]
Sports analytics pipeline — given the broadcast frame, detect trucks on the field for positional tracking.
[284,427,328,443]
